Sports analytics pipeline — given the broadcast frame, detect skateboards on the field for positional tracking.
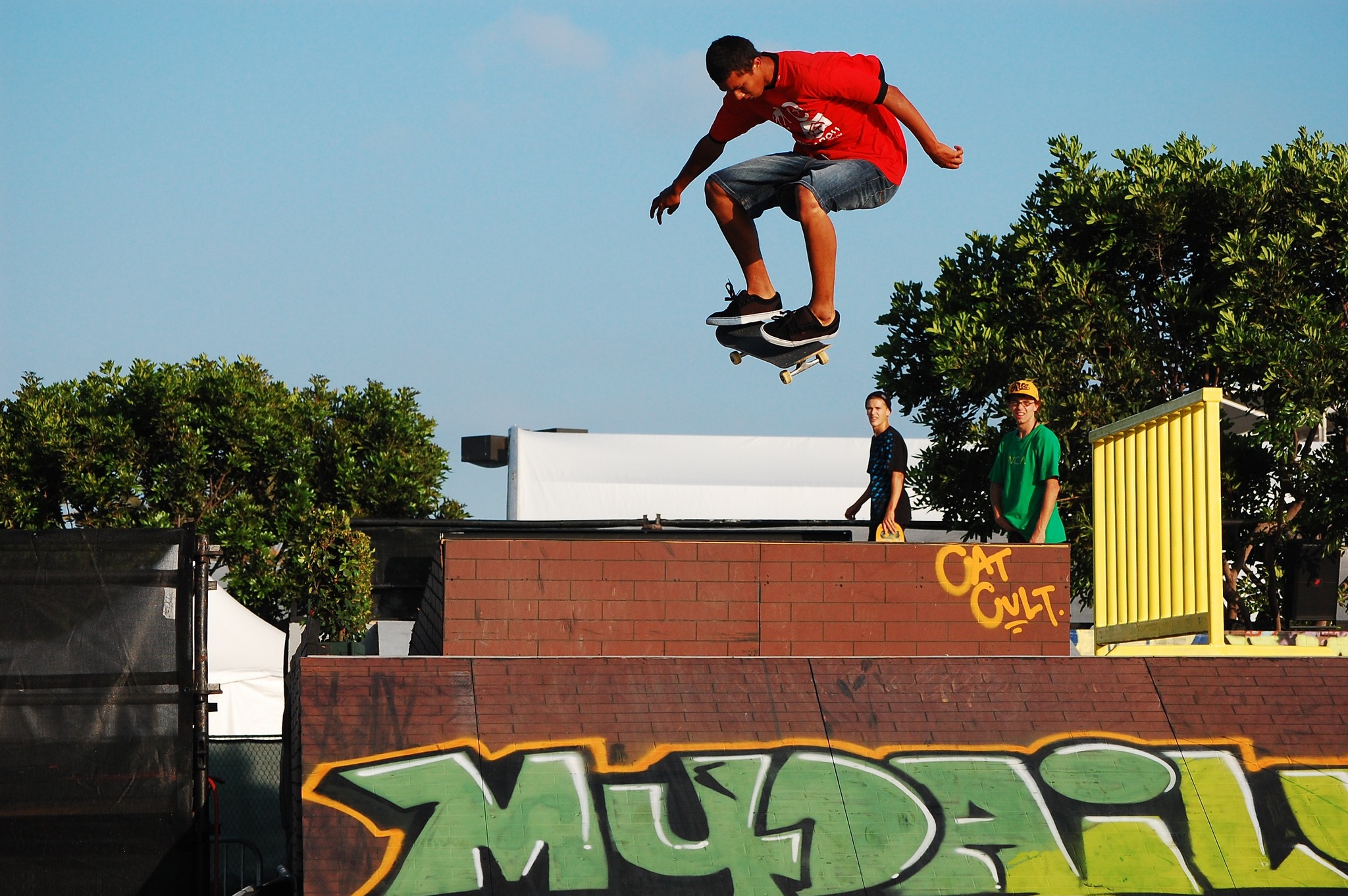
[876,521,904,542]
[715,321,832,384]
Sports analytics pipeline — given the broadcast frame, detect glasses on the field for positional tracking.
[1009,401,1035,407]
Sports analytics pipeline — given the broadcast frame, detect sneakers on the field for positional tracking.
[760,305,840,348]
[706,279,783,327]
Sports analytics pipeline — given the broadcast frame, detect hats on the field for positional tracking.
[1005,379,1039,403]
[866,391,892,413]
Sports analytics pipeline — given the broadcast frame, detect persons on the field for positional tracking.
[845,391,912,542]
[988,381,1068,545]
[650,35,963,347]
